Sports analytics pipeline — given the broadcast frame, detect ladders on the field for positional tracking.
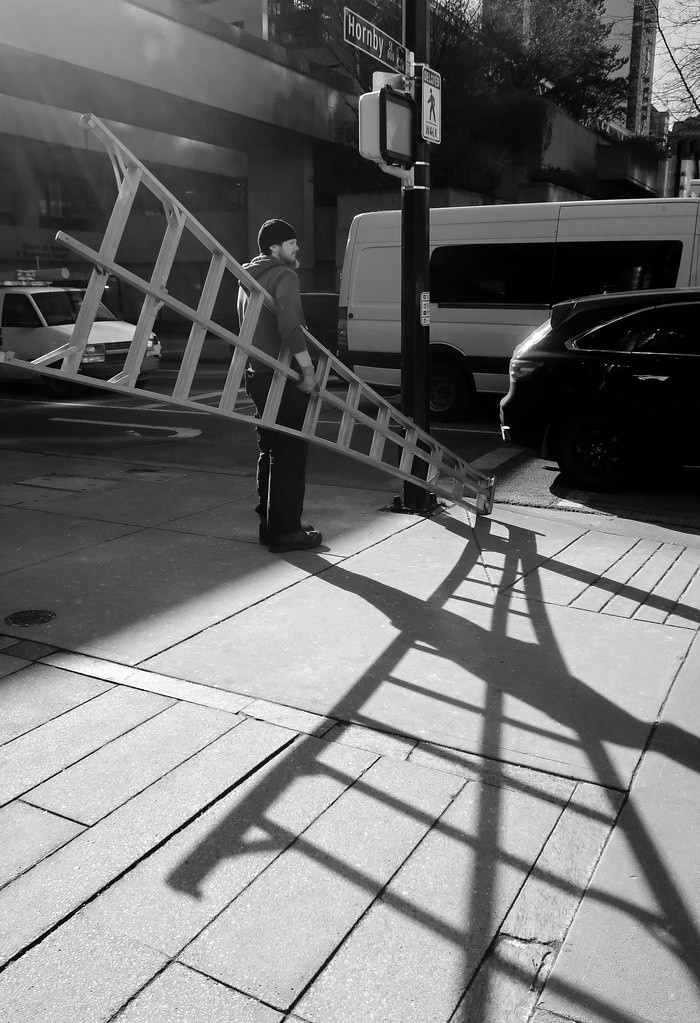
[0,114,497,517]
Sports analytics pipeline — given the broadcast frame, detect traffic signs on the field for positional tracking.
[343,6,411,78]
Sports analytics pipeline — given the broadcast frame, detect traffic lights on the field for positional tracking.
[358,84,418,167]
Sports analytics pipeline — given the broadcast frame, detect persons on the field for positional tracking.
[237,219,324,552]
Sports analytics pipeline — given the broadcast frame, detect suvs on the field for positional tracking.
[499,286,700,491]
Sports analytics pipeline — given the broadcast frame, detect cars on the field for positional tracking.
[0,279,163,399]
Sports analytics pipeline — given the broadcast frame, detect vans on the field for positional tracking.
[339,197,700,421]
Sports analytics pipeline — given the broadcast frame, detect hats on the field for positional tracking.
[258,219,297,252]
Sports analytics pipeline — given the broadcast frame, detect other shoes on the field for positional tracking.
[268,531,322,551]
[259,525,314,544]
